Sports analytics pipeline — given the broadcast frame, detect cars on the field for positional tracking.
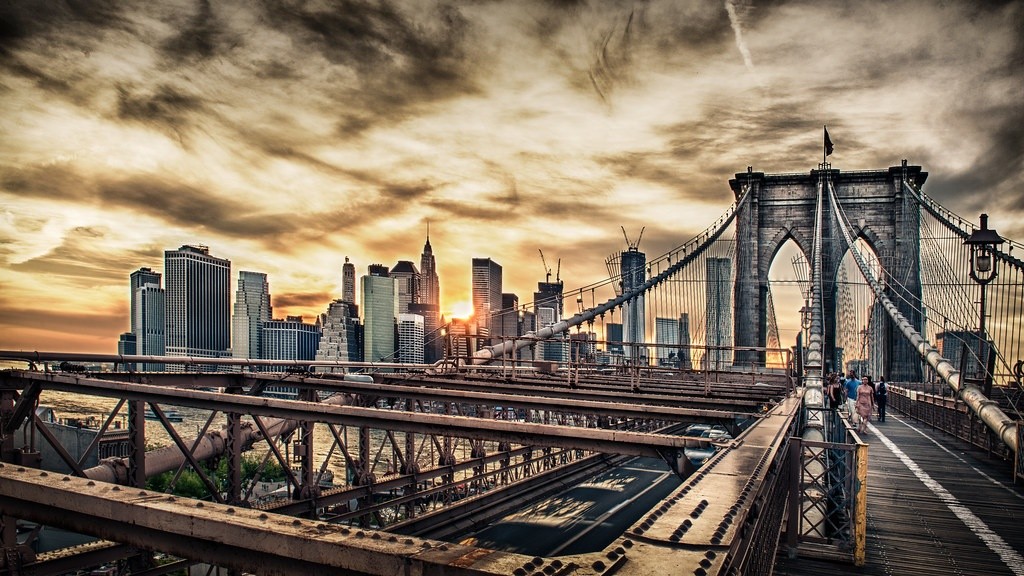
[681,421,750,470]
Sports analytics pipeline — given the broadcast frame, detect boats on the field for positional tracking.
[143,408,186,423]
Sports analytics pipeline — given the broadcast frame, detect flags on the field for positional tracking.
[824,128,834,156]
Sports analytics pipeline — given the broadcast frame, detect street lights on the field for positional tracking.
[962,212,1006,380]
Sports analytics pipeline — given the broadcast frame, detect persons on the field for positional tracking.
[826,371,862,421]
[875,375,888,423]
[864,375,876,411]
[854,377,876,434]
[846,374,863,430]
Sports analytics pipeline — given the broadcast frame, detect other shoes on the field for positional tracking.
[877,418,886,422]
[848,416,852,421]
[853,423,858,429]
[857,429,868,435]
